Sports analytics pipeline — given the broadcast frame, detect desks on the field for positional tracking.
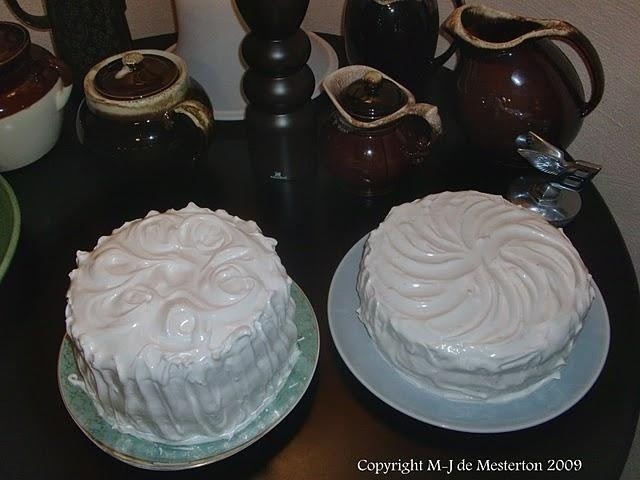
[1,118,639,480]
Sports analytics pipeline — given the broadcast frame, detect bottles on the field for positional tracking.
[2,21,74,174]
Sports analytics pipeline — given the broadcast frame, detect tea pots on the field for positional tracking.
[320,1,605,197]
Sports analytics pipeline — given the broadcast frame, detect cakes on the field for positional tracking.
[356,190,596,404]
[63,201,301,447]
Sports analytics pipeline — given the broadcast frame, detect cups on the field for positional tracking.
[76,49,215,166]
